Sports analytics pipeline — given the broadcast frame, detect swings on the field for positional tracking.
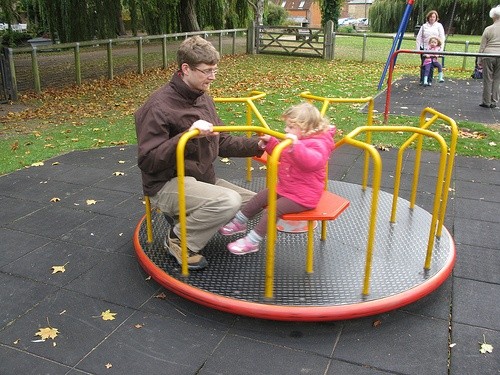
[420,0,458,62]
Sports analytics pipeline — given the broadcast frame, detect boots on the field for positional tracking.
[424,76,432,86]
[438,71,445,83]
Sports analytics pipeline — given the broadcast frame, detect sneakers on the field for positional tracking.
[164,229,208,269]
[218,218,248,235]
[164,214,179,239]
[226,236,260,255]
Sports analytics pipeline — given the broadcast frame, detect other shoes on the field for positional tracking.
[479,104,496,108]
[429,82,432,85]
[419,81,423,86]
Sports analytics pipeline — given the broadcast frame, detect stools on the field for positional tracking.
[278,187,350,272]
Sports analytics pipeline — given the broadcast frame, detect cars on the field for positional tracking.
[337,17,369,26]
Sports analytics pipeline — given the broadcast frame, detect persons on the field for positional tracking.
[221,104,337,255]
[417,11,444,85]
[478,5,500,108]
[135,36,264,268]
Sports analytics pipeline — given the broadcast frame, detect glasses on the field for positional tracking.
[188,63,219,76]
